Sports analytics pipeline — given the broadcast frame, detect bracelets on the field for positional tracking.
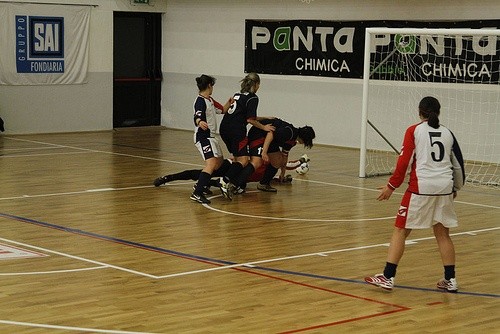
[198,119,203,125]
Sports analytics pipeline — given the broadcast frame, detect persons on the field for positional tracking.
[364,96,466,291]
[154,71,316,204]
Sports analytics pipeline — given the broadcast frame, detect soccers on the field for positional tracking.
[296,162,310,175]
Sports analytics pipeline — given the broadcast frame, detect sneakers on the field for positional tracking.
[216,176,234,201]
[364,273,396,291]
[233,185,244,195]
[435,278,459,290]
[257,181,277,192]
[190,191,213,205]
[192,183,212,195]
[152,175,169,188]
